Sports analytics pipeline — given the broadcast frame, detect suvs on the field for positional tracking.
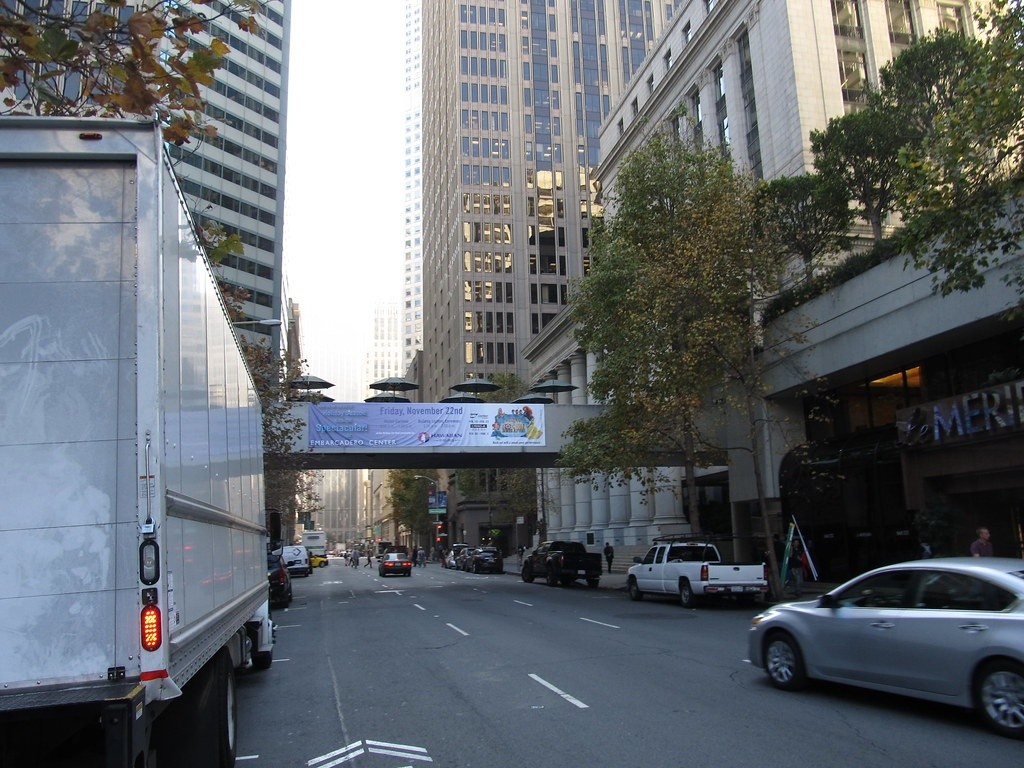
[466,547,505,574]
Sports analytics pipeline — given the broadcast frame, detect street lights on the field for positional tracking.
[414,474,439,562]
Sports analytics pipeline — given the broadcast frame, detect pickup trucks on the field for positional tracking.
[521,540,603,588]
[624,533,770,609]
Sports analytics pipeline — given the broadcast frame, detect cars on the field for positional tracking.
[377,553,412,577]
[330,543,353,556]
[443,543,476,571]
[268,555,293,607]
[310,554,329,568]
[745,555,1024,738]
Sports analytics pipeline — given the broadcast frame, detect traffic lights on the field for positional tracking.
[437,525,442,530]
[436,538,440,542]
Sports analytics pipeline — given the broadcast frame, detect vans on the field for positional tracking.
[375,540,409,564]
[281,546,313,577]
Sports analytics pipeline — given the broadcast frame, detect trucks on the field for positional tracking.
[0,114,283,766]
[303,531,327,556]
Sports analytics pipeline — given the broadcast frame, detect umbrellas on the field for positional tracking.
[286,376,335,402]
[364,377,420,402]
[510,380,580,404]
[438,378,502,403]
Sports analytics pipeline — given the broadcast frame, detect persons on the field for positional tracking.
[914,531,936,560]
[970,527,992,557]
[471,545,481,574]
[514,543,532,562]
[411,545,435,568]
[441,546,454,569]
[344,548,373,569]
[604,542,614,573]
[771,533,809,597]
